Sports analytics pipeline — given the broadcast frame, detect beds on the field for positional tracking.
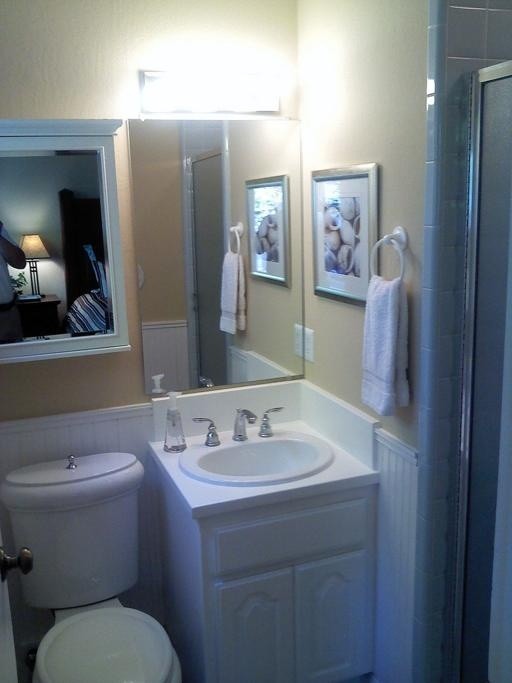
[58,187,108,333]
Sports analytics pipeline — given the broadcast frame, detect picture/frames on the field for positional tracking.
[311,163,378,301]
[245,175,291,290]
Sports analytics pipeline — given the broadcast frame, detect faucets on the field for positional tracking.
[233,405,257,440]
[196,373,213,389]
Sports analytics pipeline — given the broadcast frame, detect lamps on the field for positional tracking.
[18,233,52,297]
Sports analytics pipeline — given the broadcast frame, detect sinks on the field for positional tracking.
[178,430,334,486]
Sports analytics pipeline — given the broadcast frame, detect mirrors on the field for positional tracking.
[0,119,131,366]
[129,120,304,395]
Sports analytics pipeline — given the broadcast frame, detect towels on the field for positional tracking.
[361,275,410,416]
[219,251,246,335]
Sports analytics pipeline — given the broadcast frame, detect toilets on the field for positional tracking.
[0,450,184,683]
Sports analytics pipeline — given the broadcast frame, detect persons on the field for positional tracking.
[0,222,28,345]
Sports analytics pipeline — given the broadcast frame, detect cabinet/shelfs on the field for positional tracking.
[155,463,377,681]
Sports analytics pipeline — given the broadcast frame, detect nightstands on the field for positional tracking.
[17,294,61,337]
